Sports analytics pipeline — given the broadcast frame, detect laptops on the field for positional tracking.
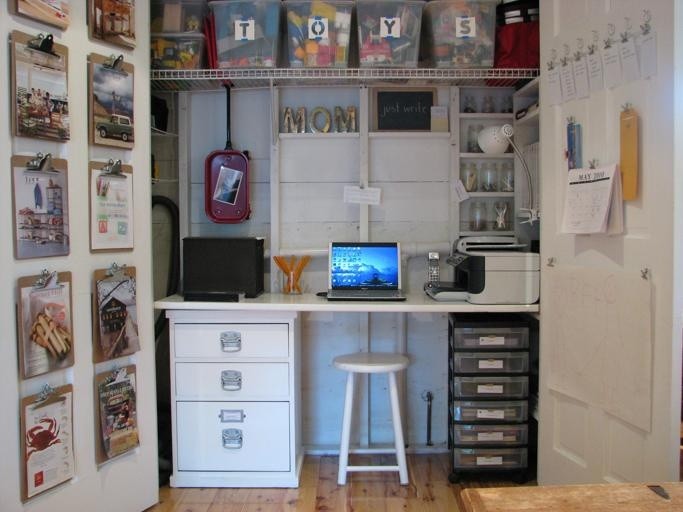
[328,242,406,301]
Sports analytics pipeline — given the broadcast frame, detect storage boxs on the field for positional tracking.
[425,0,497,68]
[279,0,356,71]
[149,27,208,71]
[207,0,281,70]
[351,0,425,69]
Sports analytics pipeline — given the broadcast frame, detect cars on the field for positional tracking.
[96,115,134,143]
[103,393,130,421]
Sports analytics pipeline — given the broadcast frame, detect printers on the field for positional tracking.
[424,237,540,305]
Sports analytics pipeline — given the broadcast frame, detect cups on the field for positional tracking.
[282,271,305,295]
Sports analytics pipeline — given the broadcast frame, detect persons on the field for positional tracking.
[30,87,54,128]
[111,90,119,115]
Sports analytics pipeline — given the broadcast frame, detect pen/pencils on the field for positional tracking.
[273,255,311,295]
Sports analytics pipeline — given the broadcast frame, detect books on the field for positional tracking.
[9,42,71,142]
[91,1,135,53]
[10,164,71,261]
[88,168,136,255]
[94,275,141,362]
[89,62,136,150]
[11,0,73,29]
[560,165,625,237]
[16,279,76,381]
[23,390,76,499]
[96,374,142,462]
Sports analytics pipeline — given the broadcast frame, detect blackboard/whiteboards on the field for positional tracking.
[372,87,438,132]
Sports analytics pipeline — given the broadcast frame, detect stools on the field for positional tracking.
[329,351,413,488]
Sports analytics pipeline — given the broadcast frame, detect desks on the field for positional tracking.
[459,481,680,510]
[154,287,542,490]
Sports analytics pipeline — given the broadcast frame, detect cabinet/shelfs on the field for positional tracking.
[512,76,542,221]
[453,89,517,237]
[440,312,539,484]
[150,125,182,186]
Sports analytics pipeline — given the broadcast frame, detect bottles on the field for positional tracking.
[469,201,508,231]
[463,95,513,113]
[468,124,484,153]
[460,162,514,192]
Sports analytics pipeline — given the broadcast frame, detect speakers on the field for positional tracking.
[183,236,264,299]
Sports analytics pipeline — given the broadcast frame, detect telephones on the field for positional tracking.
[424,251,441,292]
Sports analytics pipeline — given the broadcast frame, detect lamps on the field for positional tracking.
[470,121,535,224]
